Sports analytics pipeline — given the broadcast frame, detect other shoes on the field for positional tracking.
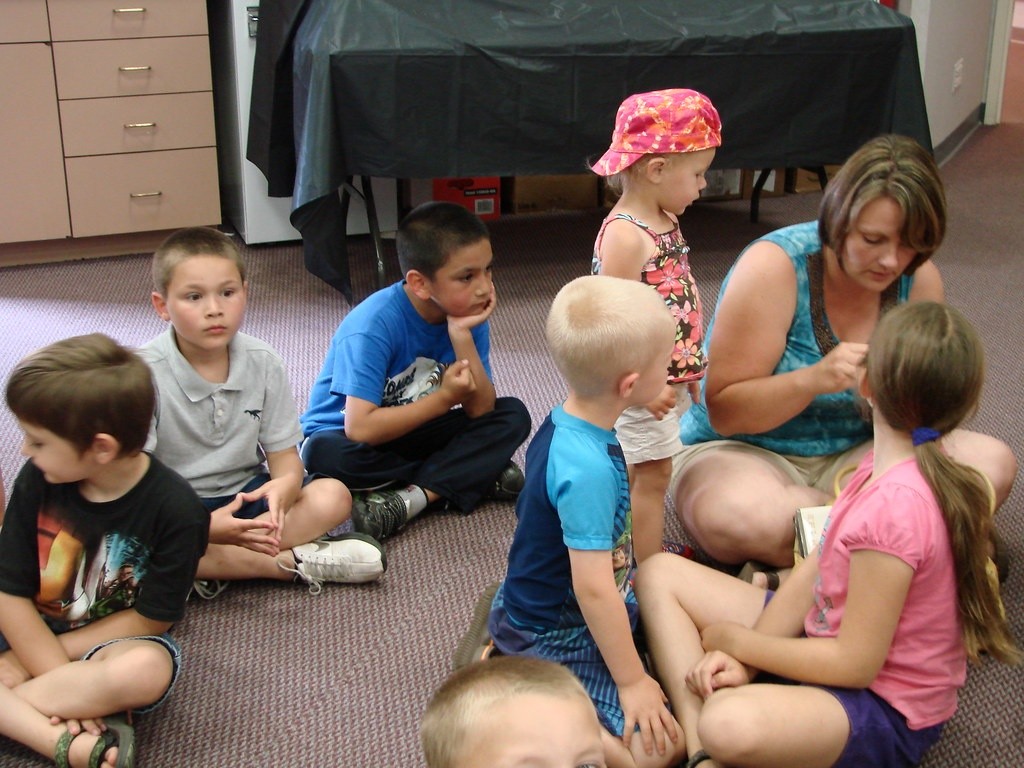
[452,582,503,670]
[736,560,780,591]
[685,750,711,768]
[631,540,697,569]
[987,527,1008,583]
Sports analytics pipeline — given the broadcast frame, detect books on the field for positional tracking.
[793,506,833,559]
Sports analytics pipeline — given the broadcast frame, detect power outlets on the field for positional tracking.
[952,57,965,94]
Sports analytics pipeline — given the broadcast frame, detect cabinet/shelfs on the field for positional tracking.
[0,0,222,267]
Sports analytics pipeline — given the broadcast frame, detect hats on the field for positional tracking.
[591,89,722,176]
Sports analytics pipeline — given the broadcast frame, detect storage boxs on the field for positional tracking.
[431,177,501,221]
[741,169,785,199]
[698,169,743,202]
[512,174,598,214]
[792,165,840,194]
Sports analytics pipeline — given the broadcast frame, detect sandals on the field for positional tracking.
[55,711,135,768]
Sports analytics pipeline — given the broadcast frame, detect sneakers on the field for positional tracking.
[352,490,406,544]
[494,462,525,499]
[277,533,387,595]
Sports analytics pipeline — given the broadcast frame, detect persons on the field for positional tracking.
[489,276,678,768]
[300,203,532,541]
[591,90,721,564]
[0,335,209,768]
[632,303,1024,768]
[420,656,607,768]
[669,135,1017,591]
[134,228,386,599]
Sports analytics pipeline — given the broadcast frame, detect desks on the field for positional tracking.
[246,0,935,306]
